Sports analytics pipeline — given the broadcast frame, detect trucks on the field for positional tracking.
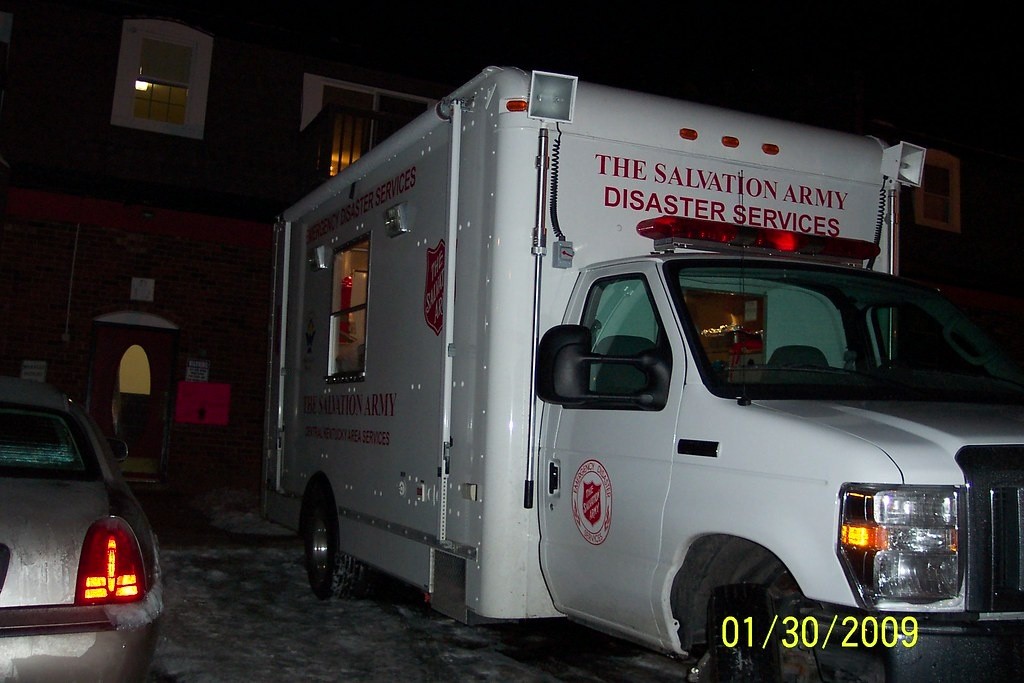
[257,66,1023,683]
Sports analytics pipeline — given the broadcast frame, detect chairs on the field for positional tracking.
[758,345,832,385]
[588,336,655,395]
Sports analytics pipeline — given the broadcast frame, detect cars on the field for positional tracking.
[0,378,163,683]
